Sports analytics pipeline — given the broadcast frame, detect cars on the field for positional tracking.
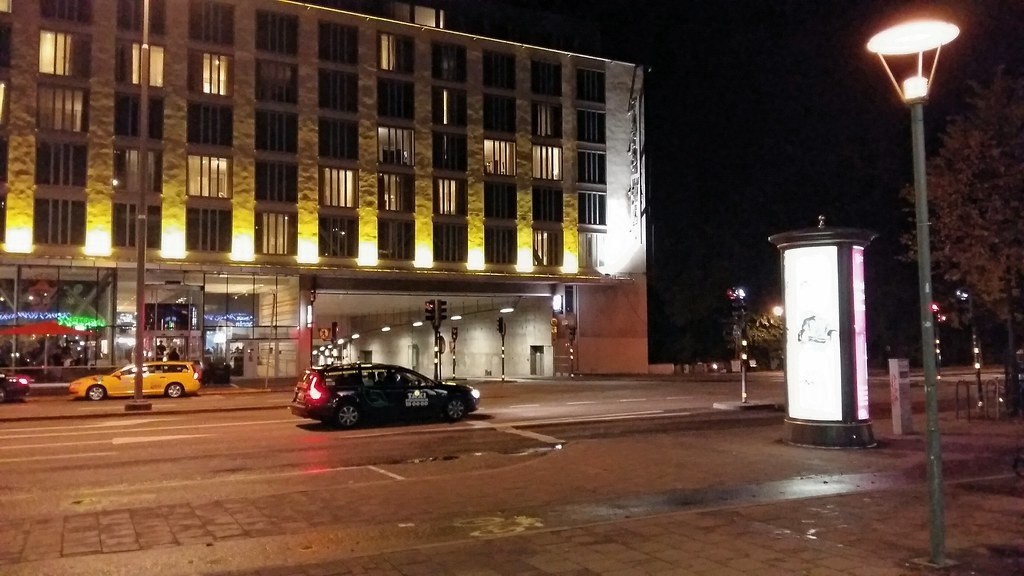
[69,360,202,402]
[0,371,31,406]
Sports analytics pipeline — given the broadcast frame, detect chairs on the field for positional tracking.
[367,371,398,388]
[335,373,359,386]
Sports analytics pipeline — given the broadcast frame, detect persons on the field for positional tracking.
[205,349,214,363]
[90,364,97,375]
[48,335,85,366]
[0,333,45,367]
[155,340,180,361]
[375,371,401,389]
[883,344,896,374]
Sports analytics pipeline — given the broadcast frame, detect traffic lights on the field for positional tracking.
[425,299,436,323]
[438,298,447,321]
[496,317,503,333]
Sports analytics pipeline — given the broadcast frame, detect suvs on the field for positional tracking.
[286,361,480,431]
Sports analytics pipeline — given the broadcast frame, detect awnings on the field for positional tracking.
[0,320,97,335]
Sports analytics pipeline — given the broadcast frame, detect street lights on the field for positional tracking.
[866,6,963,564]
